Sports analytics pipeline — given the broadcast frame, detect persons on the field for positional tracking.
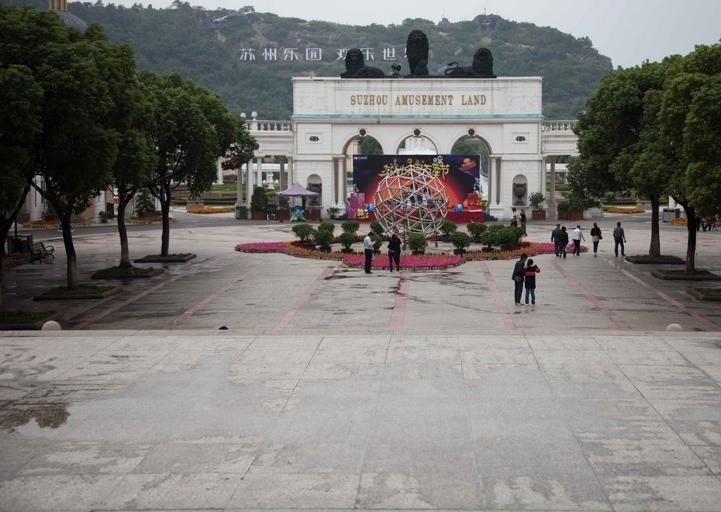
[458,155,480,185]
[384,232,404,272]
[557,226,569,258]
[613,221,628,257]
[511,252,529,306]
[510,206,522,227]
[551,223,563,258]
[519,209,529,238]
[524,258,542,307]
[398,192,428,211]
[571,224,587,257]
[591,222,604,258]
[362,232,378,275]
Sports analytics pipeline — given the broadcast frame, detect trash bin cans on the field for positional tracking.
[7,234,33,255]
[663,208,680,222]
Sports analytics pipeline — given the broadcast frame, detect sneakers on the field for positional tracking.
[524,302,536,306]
[515,302,525,306]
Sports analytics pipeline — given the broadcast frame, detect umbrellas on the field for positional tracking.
[275,181,322,206]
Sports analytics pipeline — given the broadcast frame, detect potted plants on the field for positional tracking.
[529,190,584,220]
[291,219,528,257]
[249,183,340,220]
